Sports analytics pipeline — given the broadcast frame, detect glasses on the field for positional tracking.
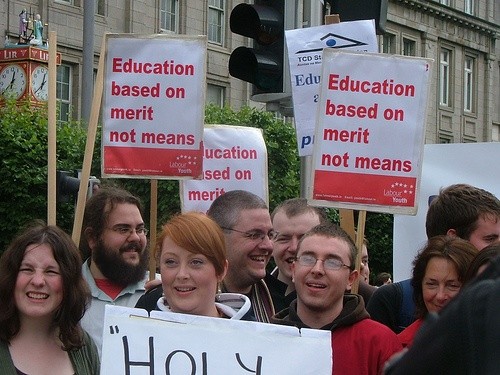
[220,226,274,242]
[103,226,150,236]
[296,255,352,269]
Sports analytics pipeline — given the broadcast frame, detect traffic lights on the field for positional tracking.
[227,0,296,104]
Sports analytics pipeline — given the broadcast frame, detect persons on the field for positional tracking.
[0,184,500,375]
[0,223,102,375]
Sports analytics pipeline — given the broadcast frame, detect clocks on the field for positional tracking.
[0,63,27,100]
[31,64,48,102]
